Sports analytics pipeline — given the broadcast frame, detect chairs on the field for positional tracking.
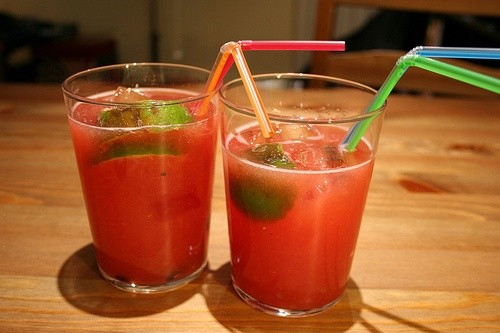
[310,0,499,99]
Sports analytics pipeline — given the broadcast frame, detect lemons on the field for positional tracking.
[236,143,295,218]
[87,99,193,166]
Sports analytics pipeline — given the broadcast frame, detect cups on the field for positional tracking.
[219,73,388,318]
[62,62,225,295]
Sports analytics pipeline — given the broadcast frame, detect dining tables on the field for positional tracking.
[0,83,500,332]
